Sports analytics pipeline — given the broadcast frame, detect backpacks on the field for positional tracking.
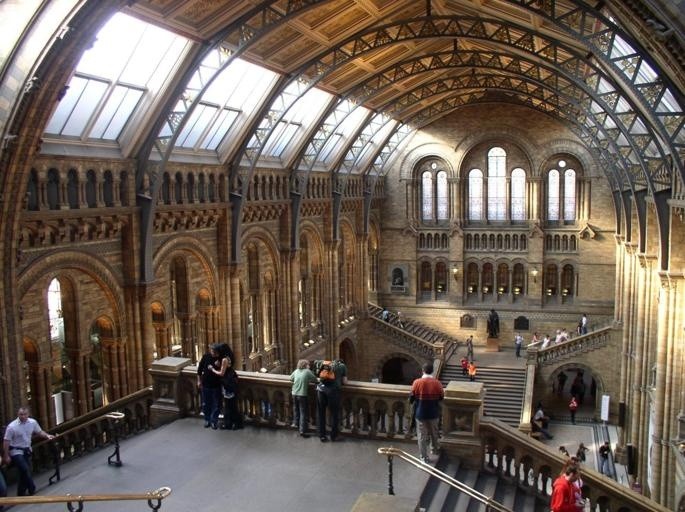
[318,360,339,380]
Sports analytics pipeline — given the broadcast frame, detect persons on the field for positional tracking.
[290,359,318,435]
[486,310,500,337]
[197,344,221,430]
[316,360,348,443]
[531,368,612,512]
[207,344,244,430]
[515,314,587,358]
[408,362,444,464]
[3,407,55,496]
[382,307,390,322]
[398,311,405,329]
[459,336,476,381]
